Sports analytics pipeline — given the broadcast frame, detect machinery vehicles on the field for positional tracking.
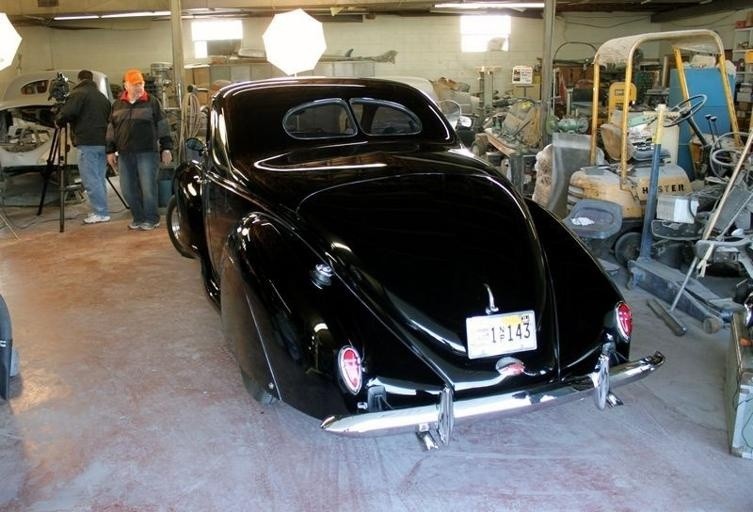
[567,29,752,334]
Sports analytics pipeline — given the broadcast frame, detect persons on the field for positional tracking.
[105,69,172,231]
[56,70,111,224]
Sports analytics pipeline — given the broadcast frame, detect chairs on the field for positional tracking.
[600,123,669,161]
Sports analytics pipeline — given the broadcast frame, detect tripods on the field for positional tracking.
[36,104,130,232]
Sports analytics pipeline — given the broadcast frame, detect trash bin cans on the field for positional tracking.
[157,166,176,208]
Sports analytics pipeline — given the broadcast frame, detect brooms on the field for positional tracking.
[644,127,753,338]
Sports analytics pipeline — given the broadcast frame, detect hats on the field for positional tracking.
[124,71,144,84]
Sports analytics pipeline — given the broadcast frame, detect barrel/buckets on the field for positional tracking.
[157,176,173,208]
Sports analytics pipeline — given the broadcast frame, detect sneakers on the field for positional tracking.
[84,213,110,222]
[128,221,160,230]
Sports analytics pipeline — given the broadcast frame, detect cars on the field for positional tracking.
[167,76,666,451]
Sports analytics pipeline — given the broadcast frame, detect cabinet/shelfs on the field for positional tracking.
[733,27,753,74]
[186,60,375,106]
